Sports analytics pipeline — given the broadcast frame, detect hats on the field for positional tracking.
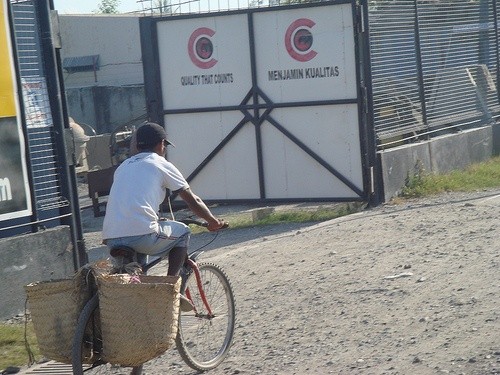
[137,121,174,147]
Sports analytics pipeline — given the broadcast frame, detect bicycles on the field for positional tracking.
[71,217,237,375]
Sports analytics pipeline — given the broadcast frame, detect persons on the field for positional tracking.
[102,123,225,312]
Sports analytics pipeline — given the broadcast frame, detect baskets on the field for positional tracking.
[96,273,182,366]
[26,278,94,363]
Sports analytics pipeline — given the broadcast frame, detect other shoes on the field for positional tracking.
[180,295,195,312]
